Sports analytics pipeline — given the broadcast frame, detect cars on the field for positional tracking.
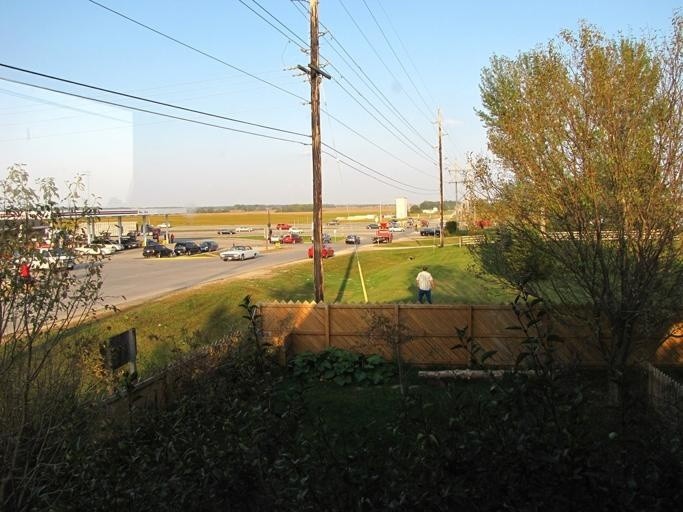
[0,224,217,268]
[219,215,440,260]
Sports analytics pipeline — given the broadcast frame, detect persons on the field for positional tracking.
[21,261,33,294]
[416,267,434,304]
